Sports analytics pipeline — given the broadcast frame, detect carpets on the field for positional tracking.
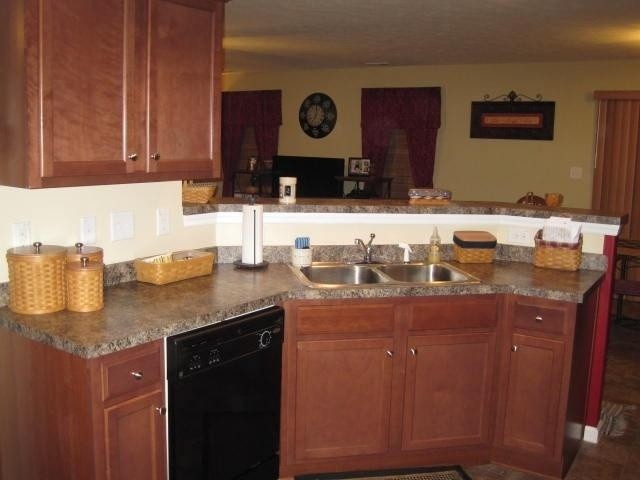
[294,464,472,480]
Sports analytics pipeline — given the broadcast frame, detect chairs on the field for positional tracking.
[613,240,640,322]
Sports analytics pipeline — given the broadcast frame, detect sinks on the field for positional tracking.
[376,259,482,288]
[285,260,390,290]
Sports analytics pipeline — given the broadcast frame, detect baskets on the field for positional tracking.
[182,179,217,204]
[534,230,583,271]
[454,231,497,264]
[134,251,214,285]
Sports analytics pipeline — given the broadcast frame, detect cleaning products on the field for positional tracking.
[427,226,441,263]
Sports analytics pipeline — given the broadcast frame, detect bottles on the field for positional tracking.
[278,176,297,204]
[246,157,259,195]
[428,227,443,263]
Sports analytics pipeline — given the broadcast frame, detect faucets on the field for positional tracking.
[354,238,373,264]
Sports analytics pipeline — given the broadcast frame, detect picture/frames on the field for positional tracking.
[349,157,373,177]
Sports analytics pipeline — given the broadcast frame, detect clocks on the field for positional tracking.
[299,92,338,138]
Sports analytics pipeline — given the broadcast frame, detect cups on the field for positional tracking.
[291,247,313,267]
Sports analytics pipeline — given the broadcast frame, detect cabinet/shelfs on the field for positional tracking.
[1,0,225,189]
[1,323,169,480]
[494,288,600,480]
[279,293,498,480]
